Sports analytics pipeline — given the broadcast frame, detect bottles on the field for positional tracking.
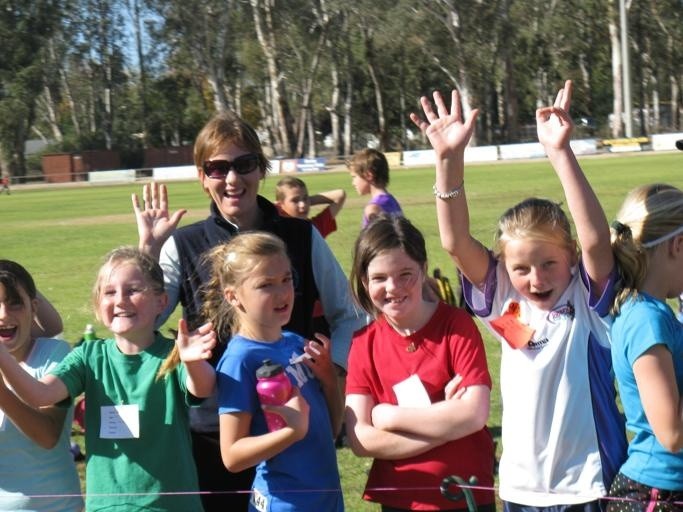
[256,358,293,432]
[83,324,96,343]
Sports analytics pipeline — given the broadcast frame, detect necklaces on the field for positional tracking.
[387,313,429,354]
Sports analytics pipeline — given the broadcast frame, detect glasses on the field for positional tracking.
[203,153,259,179]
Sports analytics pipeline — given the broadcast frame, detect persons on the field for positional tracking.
[267,176,346,240]
[1,245,222,510]
[196,231,349,507]
[342,148,404,226]
[128,110,369,510]
[340,214,498,510]
[27,293,65,340]
[406,77,636,510]
[592,180,683,511]
[0,257,89,511]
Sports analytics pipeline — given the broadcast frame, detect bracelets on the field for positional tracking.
[431,181,465,202]
